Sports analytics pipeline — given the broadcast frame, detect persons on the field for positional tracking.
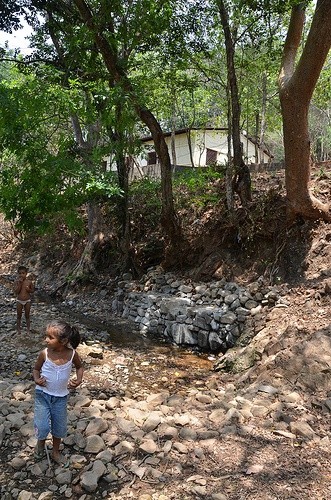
[14,266,34,335]
[33,321,83,464]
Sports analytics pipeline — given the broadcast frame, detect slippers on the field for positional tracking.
[50,451,69,468]
[34,441,45,458]
